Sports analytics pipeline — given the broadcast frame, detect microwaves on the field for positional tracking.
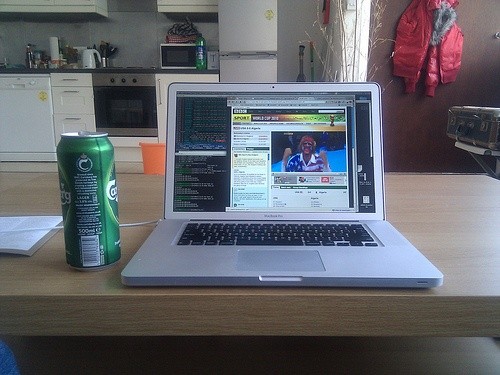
[160,44,197,69]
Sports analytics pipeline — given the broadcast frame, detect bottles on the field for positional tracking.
[196,33,208,70]
[24,46,34,69]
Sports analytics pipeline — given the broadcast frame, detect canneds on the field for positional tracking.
[55,131,122,271]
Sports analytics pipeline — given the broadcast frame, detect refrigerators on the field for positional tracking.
[218,0,277,83]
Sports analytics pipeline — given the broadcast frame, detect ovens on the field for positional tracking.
[92,72,159,137]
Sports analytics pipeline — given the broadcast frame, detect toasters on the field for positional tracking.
[207,52,219,70]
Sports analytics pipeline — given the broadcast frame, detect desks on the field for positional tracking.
[1,171,500,337]
[455,142,500,181]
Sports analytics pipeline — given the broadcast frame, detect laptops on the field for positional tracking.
[121,83,443,288]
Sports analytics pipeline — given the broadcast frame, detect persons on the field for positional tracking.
[281,135,332,173]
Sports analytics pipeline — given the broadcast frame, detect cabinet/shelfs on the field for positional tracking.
[155,74,219,143]
[157,0,219,14]
[50,73,96,147]
[0,0,108,17]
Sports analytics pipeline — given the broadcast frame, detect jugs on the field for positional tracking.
[80,49,101,69]
